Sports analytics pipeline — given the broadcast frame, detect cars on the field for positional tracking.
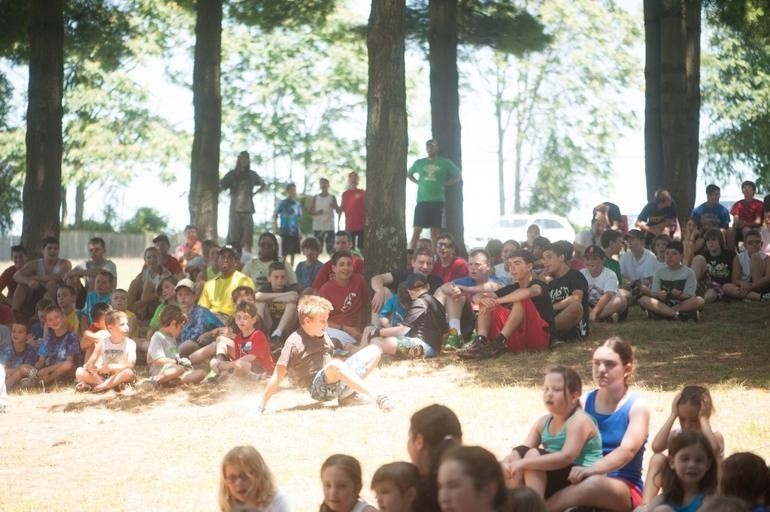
[492,212,577,246]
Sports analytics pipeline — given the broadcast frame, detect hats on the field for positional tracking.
[220,245,240,260]
[584,245,604,258]
[174,278,195,294]
[406,274,428,290]
[623,229,644,240]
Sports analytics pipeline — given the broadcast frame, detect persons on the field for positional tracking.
[408,140,462,252]
[504,336,650,512]
[633,385,770,512]
[218,151,367,250]
[371,404,550,512]
[361,225,589,358]
[683,181,770,300]
[319,454,376,512]
[572,189,705,322]
[0,235,139,392]
[219,445,289,512]
[128,224,395,411]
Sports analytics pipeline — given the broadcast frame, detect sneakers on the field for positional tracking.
[760,292,770,301]
[678,311,700,319]
[444,329,464,349]
[456,334,507,359]
